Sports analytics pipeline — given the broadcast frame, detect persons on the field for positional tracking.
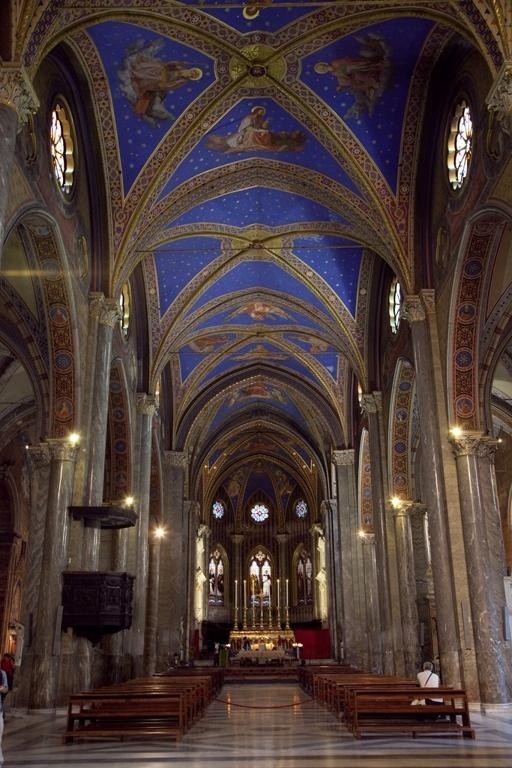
[227,108,269,149]
[211,642,225,667]
[317,58,384,93]
[129,60,199,102]
[0,669,9,766]
[1,653,16,691]
[415,662,444,705]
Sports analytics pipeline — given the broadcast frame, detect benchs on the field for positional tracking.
[62,666,227,747]
[295,664,476,741]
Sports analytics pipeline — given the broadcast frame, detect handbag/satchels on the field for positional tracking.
[411,698,426,706]
